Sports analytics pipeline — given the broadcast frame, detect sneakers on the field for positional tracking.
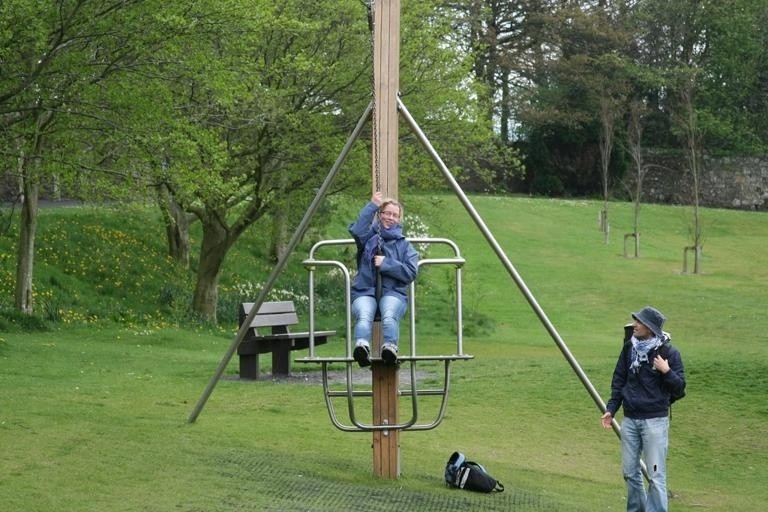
[353,345,371,367]
[381,347,397,366]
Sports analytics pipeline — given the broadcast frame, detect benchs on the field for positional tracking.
[237,299,339,379]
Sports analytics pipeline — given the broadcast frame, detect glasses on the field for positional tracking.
[379,211,399,219]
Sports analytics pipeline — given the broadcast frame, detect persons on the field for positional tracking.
[348,192,420,366]
[601,306,685,512]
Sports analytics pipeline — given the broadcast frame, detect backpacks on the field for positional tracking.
[445,451,504,493]
[624,324,686,404]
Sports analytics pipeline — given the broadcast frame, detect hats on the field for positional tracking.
[631,307,667,337]
